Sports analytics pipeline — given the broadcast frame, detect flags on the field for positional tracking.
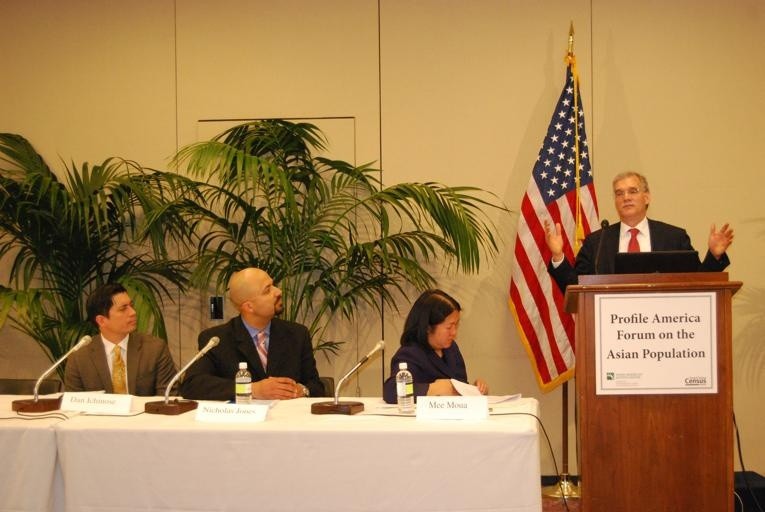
[508,52,602,393]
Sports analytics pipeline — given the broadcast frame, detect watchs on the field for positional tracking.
[297,383,310,397]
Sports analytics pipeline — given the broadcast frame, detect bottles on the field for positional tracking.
[396,363,415,411]
[235,362,253,406]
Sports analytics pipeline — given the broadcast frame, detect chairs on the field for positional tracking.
[0,378,61,394]
[317,376,335,397]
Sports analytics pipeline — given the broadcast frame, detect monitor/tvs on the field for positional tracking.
[615,251,698,274]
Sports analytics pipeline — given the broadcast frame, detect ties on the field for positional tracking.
[254,328,269,374]
[626,228,639,254]
[111,344,126,396]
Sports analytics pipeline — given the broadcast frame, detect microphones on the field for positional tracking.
[145,336,221,415]
[12,335,93,412]
[594,220,609,274]
[311,340,386,415]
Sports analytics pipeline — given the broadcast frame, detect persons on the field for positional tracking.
[180,266,326,403]
[383,289,489,405]
[542,170,734,298]
[64,283,180,399]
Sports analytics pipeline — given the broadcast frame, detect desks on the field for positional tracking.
[0,395,543,512]
